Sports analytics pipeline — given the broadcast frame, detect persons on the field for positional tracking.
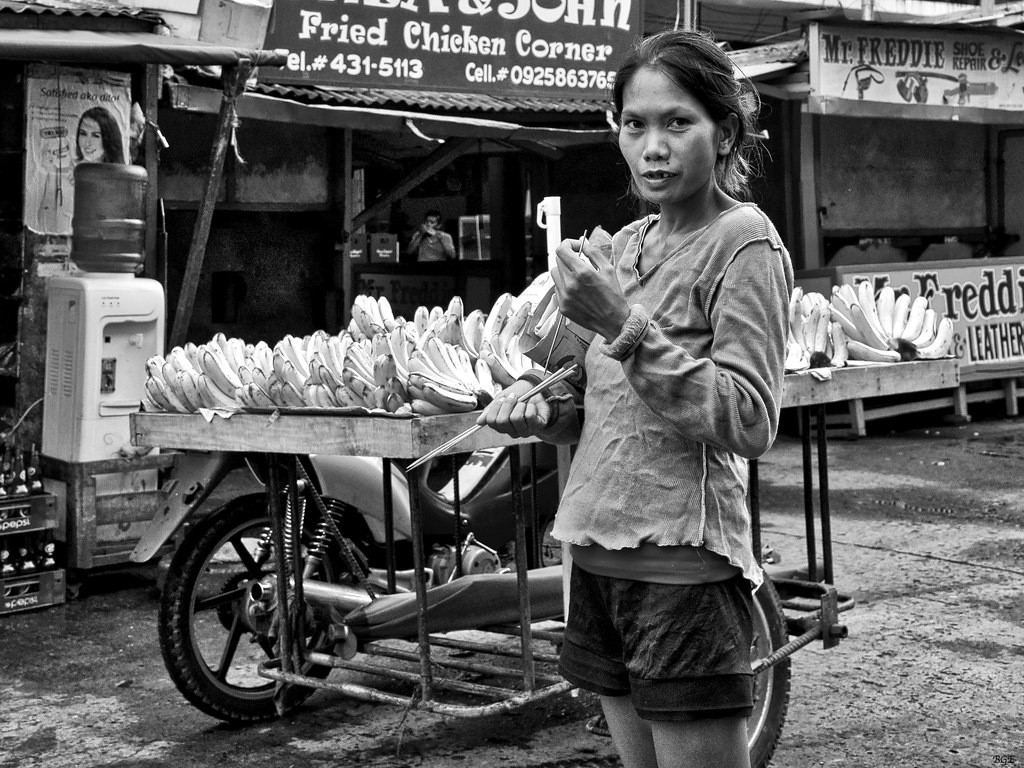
[407,209,457,263]
[74,107,125,164]
[476,29,795,768]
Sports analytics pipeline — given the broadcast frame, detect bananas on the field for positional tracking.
[135,279,955,413]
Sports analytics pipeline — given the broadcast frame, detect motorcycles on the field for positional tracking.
[129,437,582,725]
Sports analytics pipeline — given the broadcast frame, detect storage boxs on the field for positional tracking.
[0,568,65,616]
[0,491,59,535]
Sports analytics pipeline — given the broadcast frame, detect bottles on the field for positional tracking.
[0,538,56,597]
[0,443,44,519]
[70,162,147,272]
